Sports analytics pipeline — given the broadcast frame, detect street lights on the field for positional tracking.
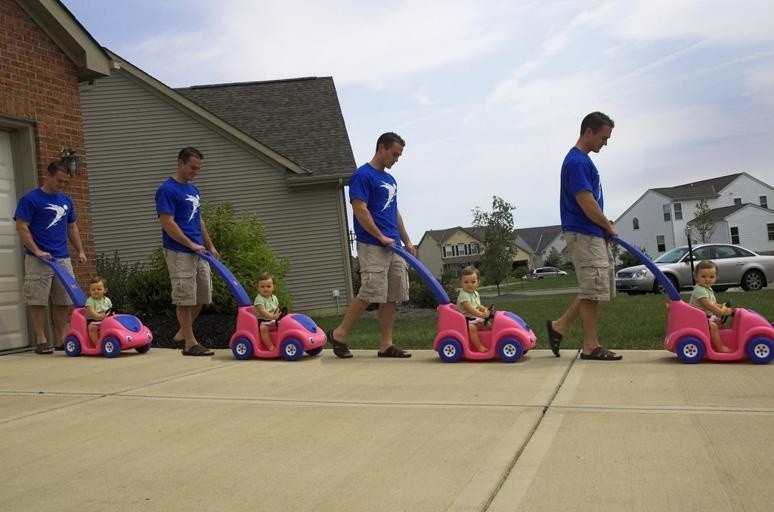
[683,224,696,286]
[686,226,697,290]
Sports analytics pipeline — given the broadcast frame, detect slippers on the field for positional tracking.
[579,347,624,361]
[376,346,410,358]
[545,319,563,356]
[35,343,55,354]
[181,344,214,356]
[326,328,352,359]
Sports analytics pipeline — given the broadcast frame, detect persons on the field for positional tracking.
[326,132,417,359]
[154,147,219,356]
[13,161,88,355]
[84,276,115,347]
[689,260,735,353]
[254,271,282,351]
[547,110,623,361]
[457,265,495,353]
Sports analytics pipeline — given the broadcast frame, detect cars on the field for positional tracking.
[527,266,568,281]
[614,242,774,296]
